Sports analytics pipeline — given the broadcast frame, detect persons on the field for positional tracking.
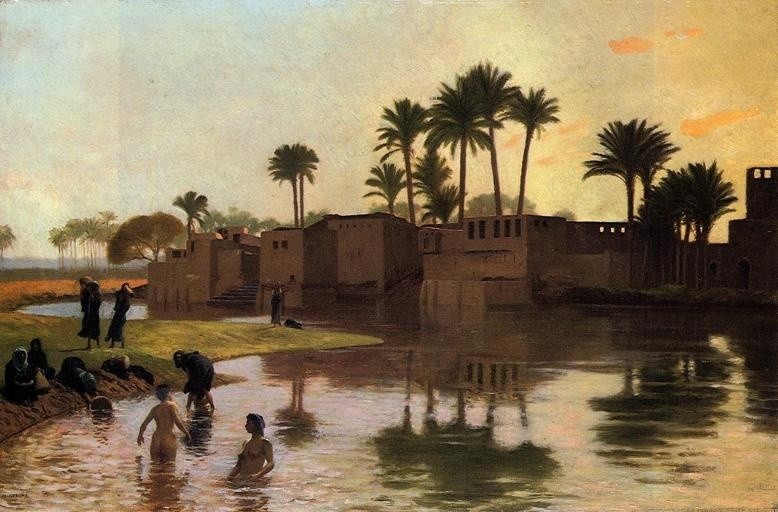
[270,293,283,327]
[2,345,40,404]
[78,280,103,349]
[55,354,106,403]
[136,383,193,458]
[183,415,216,453]
[184,374,216,415]
[24,337,54,381]
[107,281,134,349]
[173,350,215,392]
[226,412,275,480]
